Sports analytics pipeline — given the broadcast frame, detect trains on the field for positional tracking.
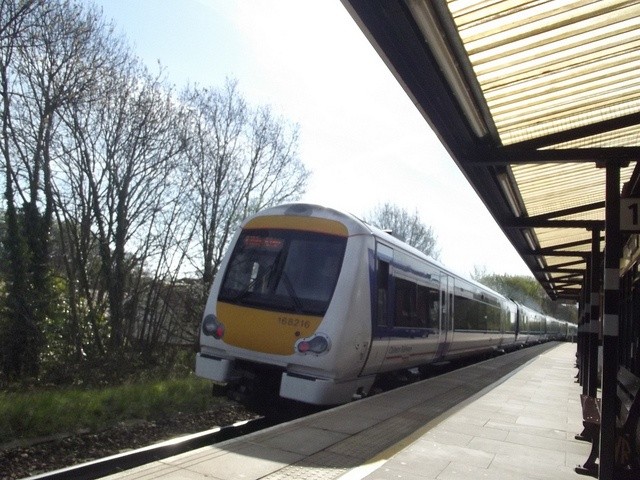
[195,204,577,419]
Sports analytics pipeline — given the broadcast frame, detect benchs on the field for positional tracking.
[573,365,640,475]
